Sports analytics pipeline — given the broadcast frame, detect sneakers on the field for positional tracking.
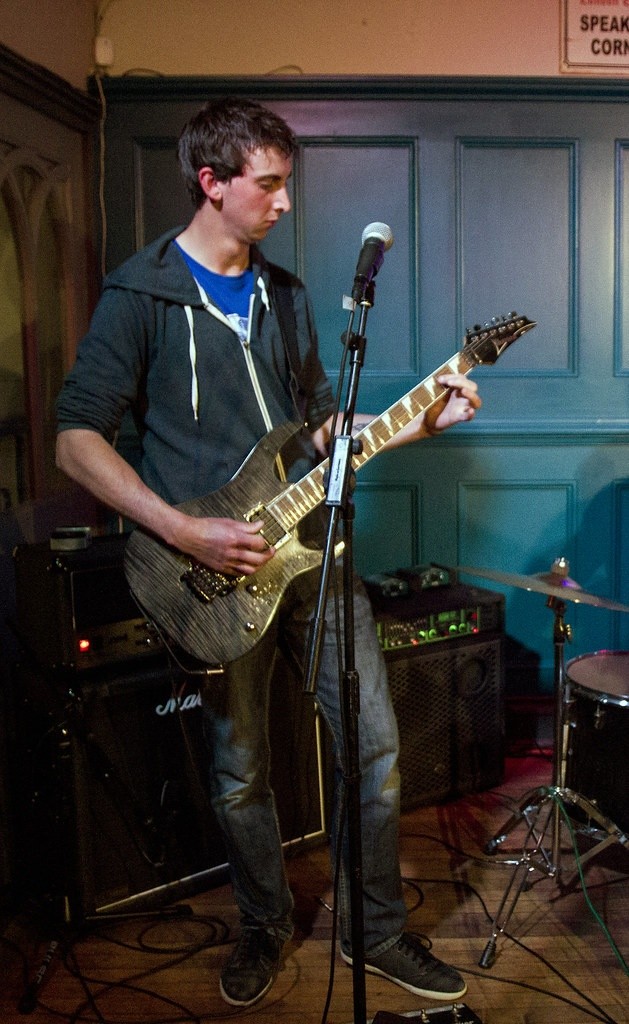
[340,931,469,1000]
[219,921,294,1006]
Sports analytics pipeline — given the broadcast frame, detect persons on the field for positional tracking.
[55,96,482,1007]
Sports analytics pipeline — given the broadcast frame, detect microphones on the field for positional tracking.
[351,222,393,302]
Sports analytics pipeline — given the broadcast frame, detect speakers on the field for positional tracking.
[22,642,330,935]
[319,582,504,829]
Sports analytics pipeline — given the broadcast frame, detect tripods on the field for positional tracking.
[0,705,193,1017]
[479,598,629,970]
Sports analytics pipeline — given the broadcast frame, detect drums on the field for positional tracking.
[561,648,629,845]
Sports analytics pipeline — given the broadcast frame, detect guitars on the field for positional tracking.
[122,310,539,665]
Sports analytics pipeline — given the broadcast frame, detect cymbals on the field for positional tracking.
[456,565,629,614]
